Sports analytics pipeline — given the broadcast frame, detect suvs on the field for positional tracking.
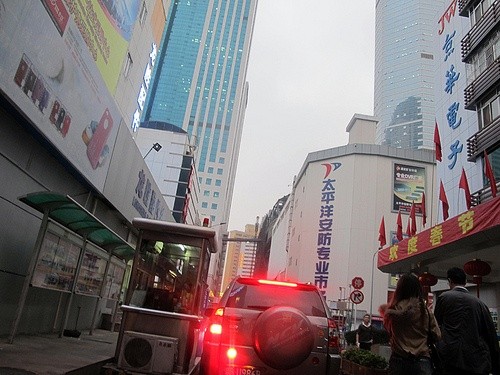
[203,274,343,375]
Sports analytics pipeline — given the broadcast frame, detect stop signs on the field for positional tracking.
[351,276,364,290]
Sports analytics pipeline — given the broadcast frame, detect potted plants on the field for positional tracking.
[342,347,389,375]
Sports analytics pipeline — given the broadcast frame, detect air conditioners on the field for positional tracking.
[117,330,179,375]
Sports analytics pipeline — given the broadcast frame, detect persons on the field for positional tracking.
[358,314,374,350]
[435,266,500,375]
[384,274,442,375]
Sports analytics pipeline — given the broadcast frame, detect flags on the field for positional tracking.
[422,193,426,226]
[378,216,386,246]
[439,181,449,221]
[484,150,497,198]
[434,122,442,162]
[397,211,403,241]
[459,168,471,210]
[406,201,416,237]
